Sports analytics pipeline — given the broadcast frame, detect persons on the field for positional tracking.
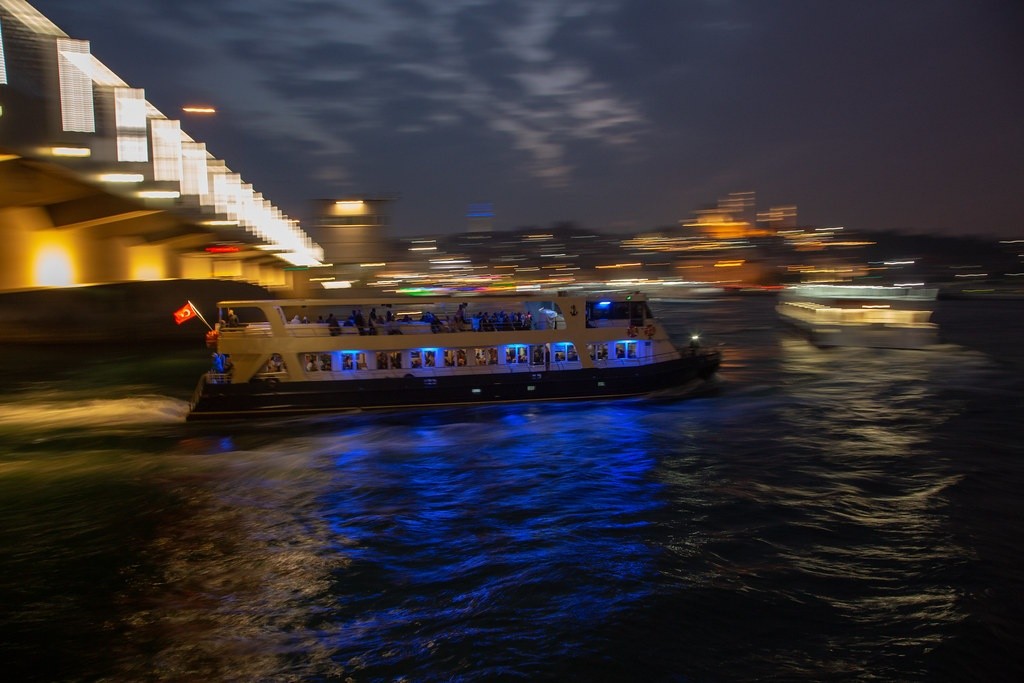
[316,301,538,336]
[219,319,227,331]
[209,350,232,384]
[264,343,636,376]
[301,316,310,323]
[227,308,239,327]
[289,314,301,324]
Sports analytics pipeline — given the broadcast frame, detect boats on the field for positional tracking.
[173,284,727,419]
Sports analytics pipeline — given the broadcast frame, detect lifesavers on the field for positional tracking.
[626,325,638,338]
[490,383,504,398]
[645,323,655,336]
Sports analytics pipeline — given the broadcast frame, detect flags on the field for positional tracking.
[173,303,196,324]
